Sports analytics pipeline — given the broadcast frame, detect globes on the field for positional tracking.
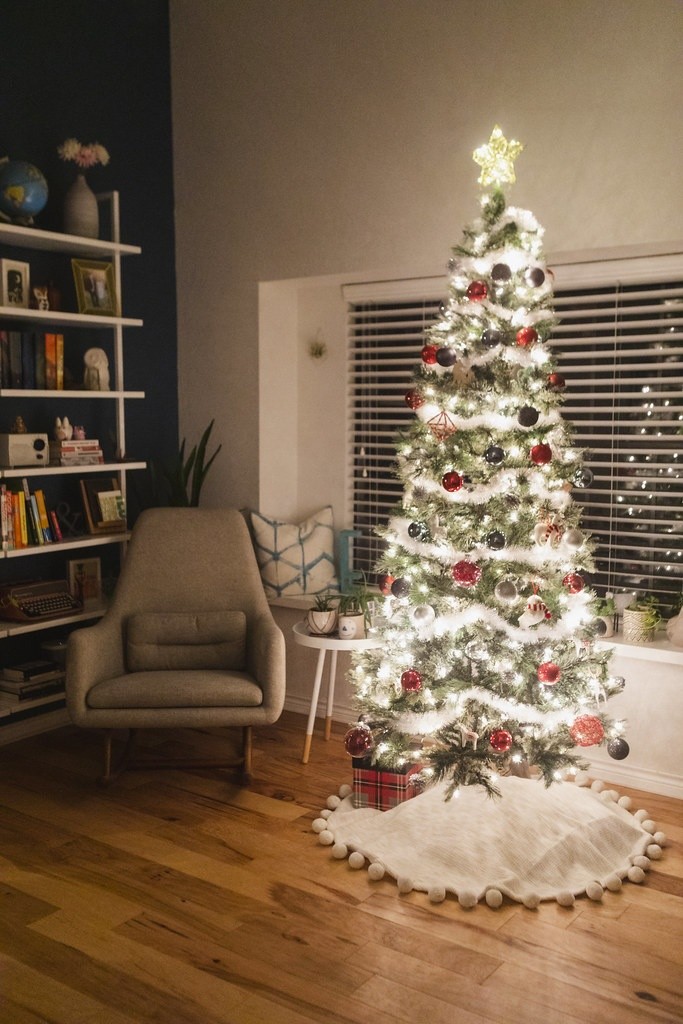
[0,156,48,228]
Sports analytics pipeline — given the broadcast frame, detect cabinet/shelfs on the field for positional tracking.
[0,190,149,720]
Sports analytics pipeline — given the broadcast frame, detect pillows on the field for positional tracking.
[249,505,342,606]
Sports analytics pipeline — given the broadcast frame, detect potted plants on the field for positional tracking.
[664,592,683,646]
[304,594,340,635]
[612,589,637,616]
[623,595,662,644]
[337,568,374,640]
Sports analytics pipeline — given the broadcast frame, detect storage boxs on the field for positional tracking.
[350,760,421,812]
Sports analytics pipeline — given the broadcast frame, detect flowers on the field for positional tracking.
[58,137,110,172]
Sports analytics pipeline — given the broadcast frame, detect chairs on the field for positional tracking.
[67,504,286,789]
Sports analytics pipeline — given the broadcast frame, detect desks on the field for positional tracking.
[293,617,386,765]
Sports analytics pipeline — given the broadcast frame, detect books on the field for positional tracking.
[47,440,107,468]
[0,476,63,553]
[0,659,66,701]
[0,329,63,390]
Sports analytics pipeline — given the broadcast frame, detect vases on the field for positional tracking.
[63,174,100,238]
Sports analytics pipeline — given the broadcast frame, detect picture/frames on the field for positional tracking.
[68,557,101,608]
[79,478,127,534]
[0,257,30,308]
[70,259,117,316]
